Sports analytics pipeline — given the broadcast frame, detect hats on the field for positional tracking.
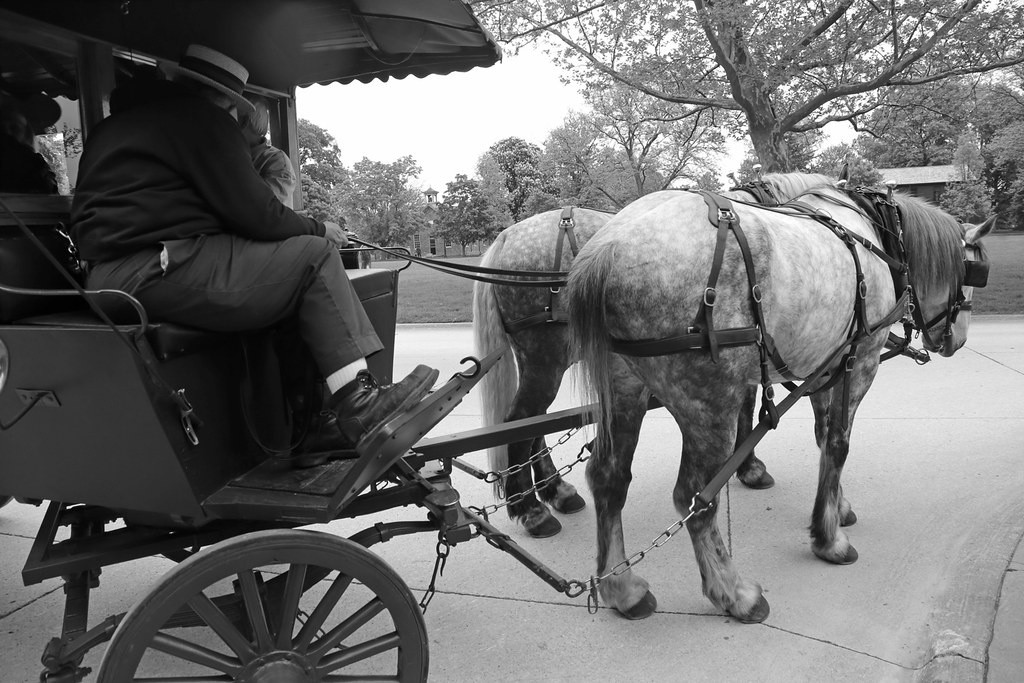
[156,43,258,115]
[0,69,62,134]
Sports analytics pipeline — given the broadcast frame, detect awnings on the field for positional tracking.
[0,0,504,91]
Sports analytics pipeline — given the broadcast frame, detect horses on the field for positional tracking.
[468,167,851,541]
[560,178,998,626]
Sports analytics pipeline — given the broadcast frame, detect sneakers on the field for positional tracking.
[329,364,440,457]
[289,404,357,468]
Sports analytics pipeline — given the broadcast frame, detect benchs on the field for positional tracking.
[0,187,411,522]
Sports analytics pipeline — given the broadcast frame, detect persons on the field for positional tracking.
[0,81,66,321]
[416,246,421,258]
[70,46,440,464]
[233,97,295,216]
